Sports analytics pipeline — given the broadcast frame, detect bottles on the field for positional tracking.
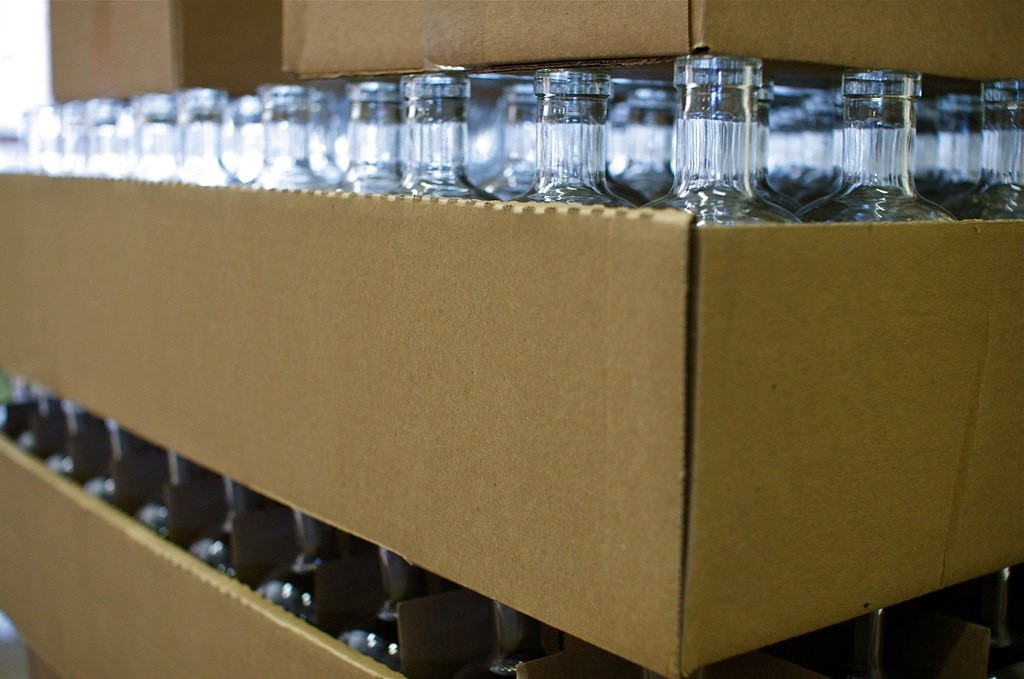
[0,56,1023,227]
[0,372,1024,679]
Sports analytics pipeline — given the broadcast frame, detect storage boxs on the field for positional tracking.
[0,0,1024,679]
